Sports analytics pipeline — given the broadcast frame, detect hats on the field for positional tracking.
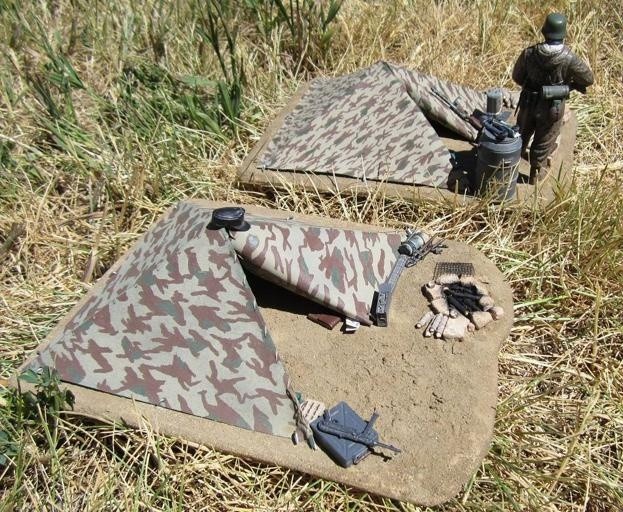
[211,207,251,232]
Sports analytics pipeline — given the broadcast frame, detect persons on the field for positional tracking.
[510,13,595,185]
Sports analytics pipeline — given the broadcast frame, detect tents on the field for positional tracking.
[21,195,407,439]
[258,58,510,194]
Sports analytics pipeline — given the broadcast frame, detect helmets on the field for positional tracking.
[541,13,567,40]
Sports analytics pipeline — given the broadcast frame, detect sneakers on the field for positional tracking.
[531,171,544,183]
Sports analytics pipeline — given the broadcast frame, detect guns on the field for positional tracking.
[373,208,447,330]
[431,86,517,143]
[311,410,407,454]
